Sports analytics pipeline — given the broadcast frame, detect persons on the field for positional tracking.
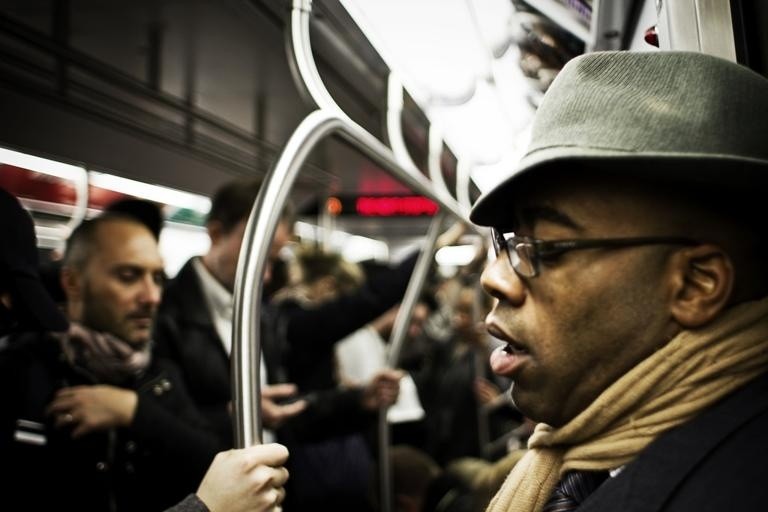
[1,175,535,511]
[482,49,767,511]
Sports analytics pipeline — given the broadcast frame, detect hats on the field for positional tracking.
[468,50,764,228]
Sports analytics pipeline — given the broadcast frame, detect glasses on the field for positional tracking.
[489,222,733,280]
